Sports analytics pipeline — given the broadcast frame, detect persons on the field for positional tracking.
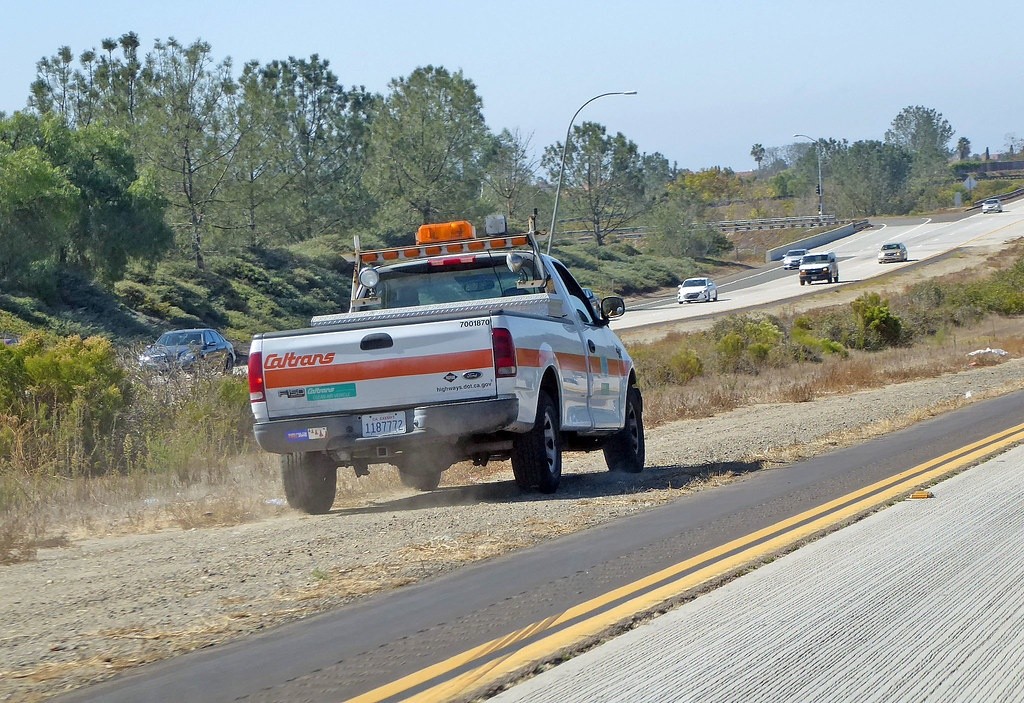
[398,287,421,307]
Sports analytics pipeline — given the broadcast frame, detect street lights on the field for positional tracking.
[793,134,824,221]
[545,89,638,256]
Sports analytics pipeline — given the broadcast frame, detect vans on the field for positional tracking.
[799,250,839,286]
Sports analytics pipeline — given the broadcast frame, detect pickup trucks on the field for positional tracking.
[243,212,647,515]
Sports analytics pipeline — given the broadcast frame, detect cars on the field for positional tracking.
[878,243,907,264]
[677,277,718,305]
[981,199,1003,214]
[138,329,235,381]
[782,248,809,271]
